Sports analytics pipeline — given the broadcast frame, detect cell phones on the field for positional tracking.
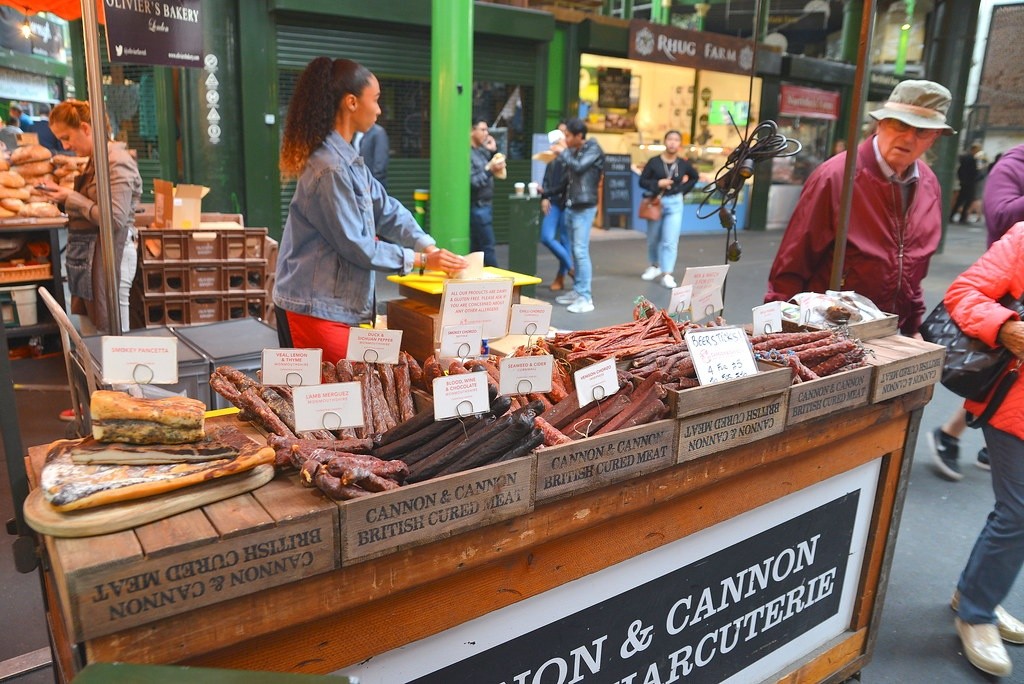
[483,139,489,145]
[33,184,51,191]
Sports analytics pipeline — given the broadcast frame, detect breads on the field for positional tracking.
[0,143,90,219]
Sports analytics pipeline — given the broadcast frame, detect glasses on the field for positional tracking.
[887,117,935,139]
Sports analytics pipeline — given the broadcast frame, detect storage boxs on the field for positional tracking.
[0,284,40,327]
[30,310,947,645]
[134,176,278,326]
[0,258,52,283]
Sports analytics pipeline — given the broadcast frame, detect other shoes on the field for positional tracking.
[952,594,1024,644]
[960,218,971,226]
[550,273,565,290]
[641,266,662,280]
[660,275,677,289]
[954,616,1013,677]
[949,216,955,223]
[568,269,575,278]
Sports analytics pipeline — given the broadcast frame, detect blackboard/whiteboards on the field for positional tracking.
[598,67,631,109]
[686,325,758,385]
[603,154,634,215]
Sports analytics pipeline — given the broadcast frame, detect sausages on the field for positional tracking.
[515,299,867,446]
[209,347,547,498]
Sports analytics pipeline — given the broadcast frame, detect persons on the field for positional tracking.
[0,101,75,169]
[536,119,575,291]
[42,98,144,421]
[944,219,1024,676]
[272,57,470,386]
[638,131,699,289]
[926,136,1024,480]
[762,80,959,344]
[469,115,507,268]
[550,117,606,313]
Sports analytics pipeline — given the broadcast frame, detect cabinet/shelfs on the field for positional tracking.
[0,216,68,339]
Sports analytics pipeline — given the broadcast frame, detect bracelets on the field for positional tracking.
[419,252,426,275]
[84,200,95,221]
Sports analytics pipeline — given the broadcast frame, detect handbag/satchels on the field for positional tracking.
[918,291,1024,403]
[640,198,661,220]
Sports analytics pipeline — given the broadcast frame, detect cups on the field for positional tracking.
[514,182,525,196]
[528,182,538,196]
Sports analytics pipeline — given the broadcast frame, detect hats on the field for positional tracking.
[867,79,958,136]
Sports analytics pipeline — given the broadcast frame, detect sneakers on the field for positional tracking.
[974,448,991,469]
[555,291,578,303]
[59,404,83,421]
[926,424,964,481]
[567,296,595,313]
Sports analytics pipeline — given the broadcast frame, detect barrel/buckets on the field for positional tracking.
[0,283,38,328]
